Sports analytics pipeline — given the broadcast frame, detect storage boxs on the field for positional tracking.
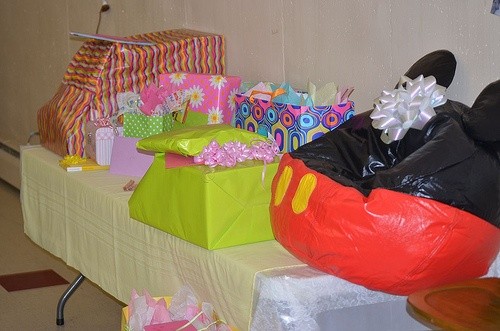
[37,28,357,251]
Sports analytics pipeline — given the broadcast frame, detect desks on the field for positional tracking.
[20,132,500,331]
